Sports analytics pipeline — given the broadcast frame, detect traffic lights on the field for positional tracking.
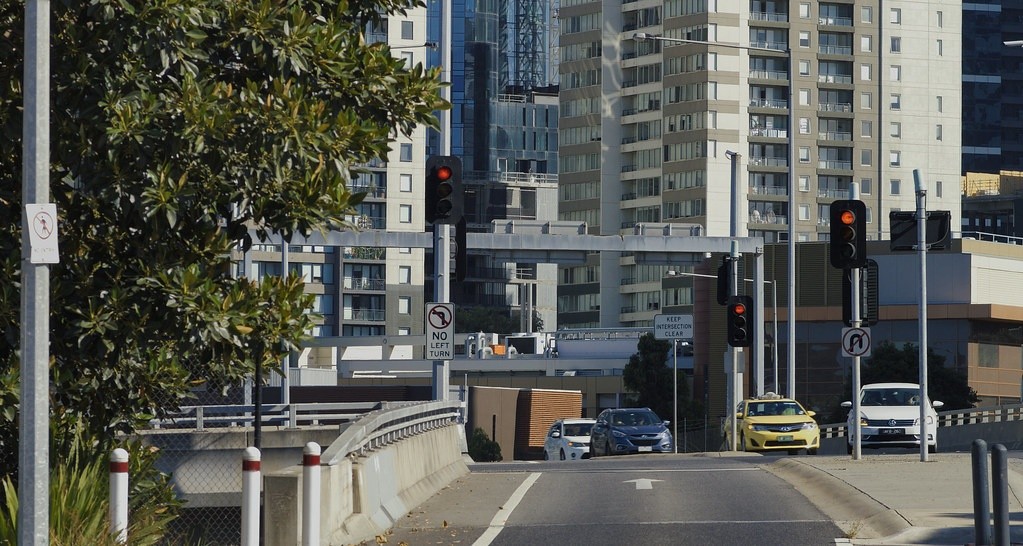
[426,155,464,225]
[829,199,867,269]
[727,295,754,347]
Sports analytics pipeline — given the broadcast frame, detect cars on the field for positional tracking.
[589,407,673,457]
[840,383,944,452]
[722,392,820,455]
[543,417,598,460]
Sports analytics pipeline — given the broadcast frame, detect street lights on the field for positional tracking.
[673,337,690,453]
[666,269,779,395]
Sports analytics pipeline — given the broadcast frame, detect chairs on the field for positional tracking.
[565,429,574,435]
